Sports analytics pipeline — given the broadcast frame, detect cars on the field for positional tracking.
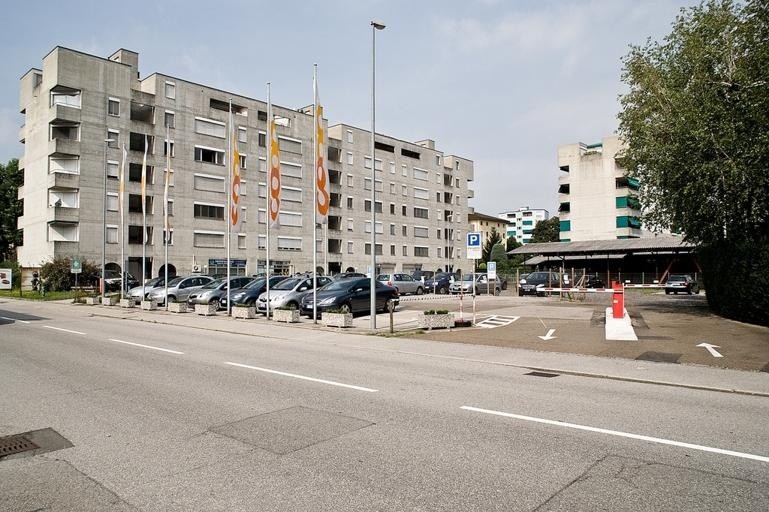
[298,276,399,319]
[92,267,140,294]
[147,276,216,307]
[333,269,605,298]
[255,275,336,316]
[663,274,701,295]
[126,275,179,306]
[218,276,293,312]
[186,277,257,311]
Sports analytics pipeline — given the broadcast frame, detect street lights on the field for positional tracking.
[446,214,454,272]
[370,21,386,330]
[100,138,116,306]
